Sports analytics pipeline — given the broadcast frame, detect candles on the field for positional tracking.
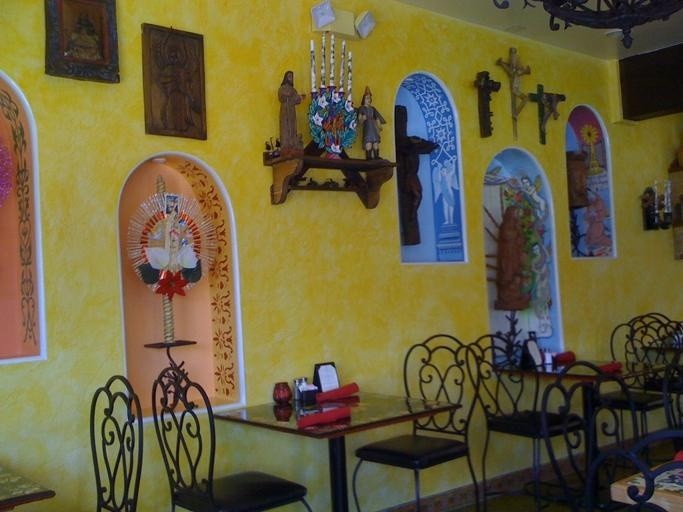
[309,29,353,102]
[652,178,674,212]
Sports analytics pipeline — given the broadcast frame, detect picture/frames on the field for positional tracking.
[43,0,120,84]
[140,23,209,143]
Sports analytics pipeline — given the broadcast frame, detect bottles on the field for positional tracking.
[300,377,308,399]
[273,382,292,402]
[539,348,544,361]
[544,348,552,364]
[295,378,300,399]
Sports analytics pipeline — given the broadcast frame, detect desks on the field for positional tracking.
[0,464,56,512]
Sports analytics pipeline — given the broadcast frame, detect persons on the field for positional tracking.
[432,157,460,228]
[357,87,389,159]
[153,46,195,132]
[496,58,531,121]
[579,186,613,257]
[278,70,306,151]
[539,93,560,136]
[402,152,423,220]
[504,175,546,217]
[529,241,554,325]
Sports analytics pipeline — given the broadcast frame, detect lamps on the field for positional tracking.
[492,0,683,48]
